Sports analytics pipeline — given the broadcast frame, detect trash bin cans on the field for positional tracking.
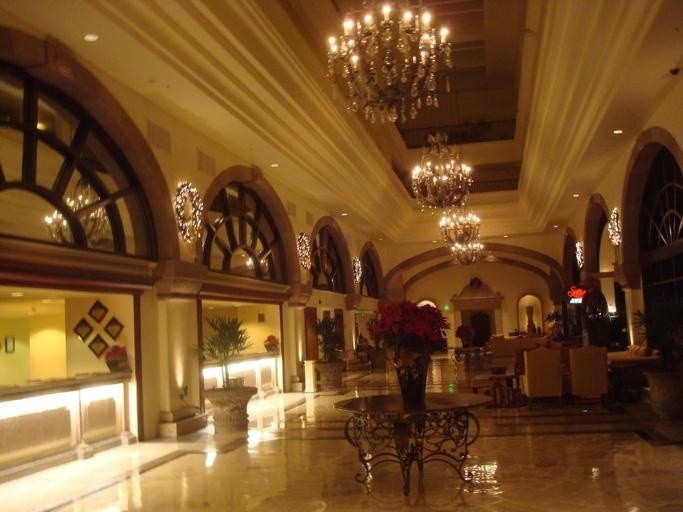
[228,378,245,387]
[303,360,318,393]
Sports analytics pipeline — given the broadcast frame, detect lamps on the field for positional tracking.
[439,206,482,266]
[242,248,269,273]
[45,186,109,247]
[411,126,476,208]
[323,0,456,126]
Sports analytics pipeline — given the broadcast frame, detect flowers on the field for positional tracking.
[371,297,449,364]
[455,325,475,337]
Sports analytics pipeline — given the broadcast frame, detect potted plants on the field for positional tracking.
[631,298,683,418]
[366,319,385,368]
[393,352,431,404]
[190,315,257,434]
[312,317,346,387]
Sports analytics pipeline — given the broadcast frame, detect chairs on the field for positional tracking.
[455,345,610,412]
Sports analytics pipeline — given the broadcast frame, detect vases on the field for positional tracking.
[462,335,472,347]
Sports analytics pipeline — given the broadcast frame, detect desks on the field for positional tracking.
[334,393,493,495]
